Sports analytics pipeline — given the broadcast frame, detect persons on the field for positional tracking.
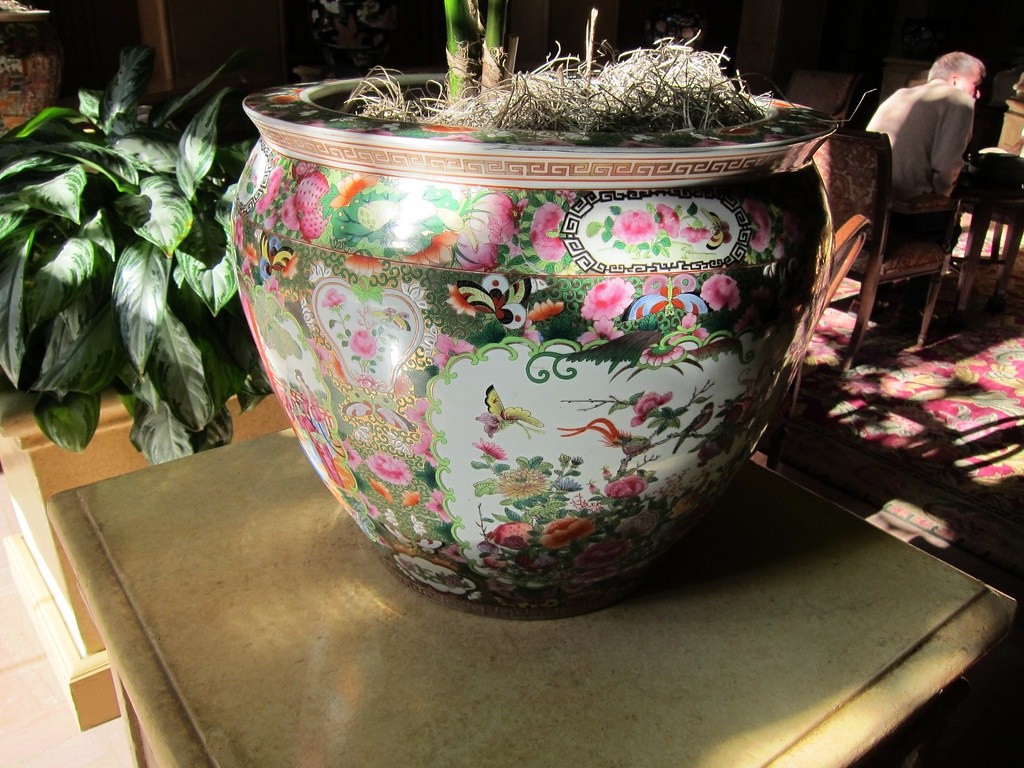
[866,51,987,331]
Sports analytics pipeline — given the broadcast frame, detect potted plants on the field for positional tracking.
[229,0,841,617]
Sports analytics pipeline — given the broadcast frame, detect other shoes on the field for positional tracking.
[899,307,939,330]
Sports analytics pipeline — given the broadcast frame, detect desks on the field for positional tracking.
[46,421,1013,766]
[958,184,1024,314]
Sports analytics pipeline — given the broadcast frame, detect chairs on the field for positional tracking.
[809,131,958,372]
